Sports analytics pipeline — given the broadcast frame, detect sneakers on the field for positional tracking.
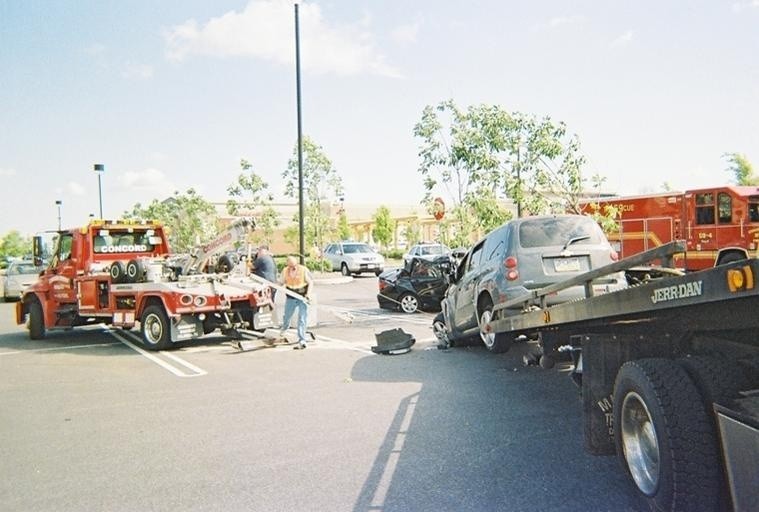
[300,341,306,348]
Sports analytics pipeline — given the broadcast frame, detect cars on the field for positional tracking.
[376,238,470,314]
[0,256,50,302]
[321,241,385,278]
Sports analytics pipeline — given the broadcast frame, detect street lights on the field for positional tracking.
[55,200,62,231]
[93,163,107,219]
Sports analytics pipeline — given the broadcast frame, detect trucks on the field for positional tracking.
[566,184,759,277]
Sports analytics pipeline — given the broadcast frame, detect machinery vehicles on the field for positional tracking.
[15,220,275,351]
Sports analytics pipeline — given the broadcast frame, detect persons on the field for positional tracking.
[271,256,312,349]
[158,250,250,282]
[246,244,278,303]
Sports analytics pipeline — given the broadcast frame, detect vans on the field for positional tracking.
[441,216,629,354]
